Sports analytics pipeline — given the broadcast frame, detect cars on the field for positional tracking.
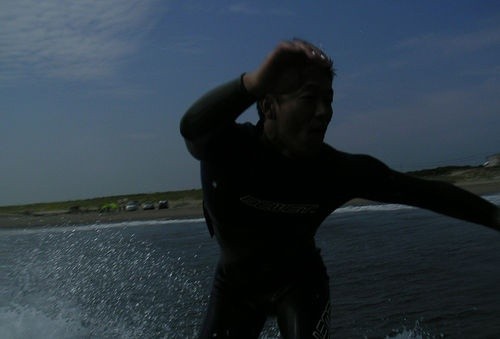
[143,201,154,210]
[126,202,138,211]
[158,200,168,210]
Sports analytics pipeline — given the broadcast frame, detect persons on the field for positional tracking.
[180,38,500,339]
